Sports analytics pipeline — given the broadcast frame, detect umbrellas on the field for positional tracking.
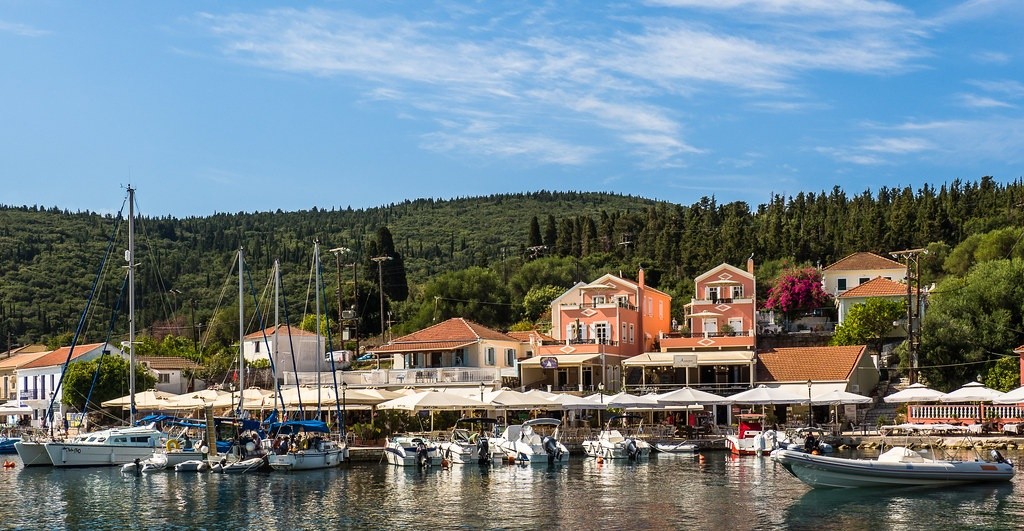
[941,381,1005,424]
[0,402,33,424]
[883,382,946,425]
[992,385,1024,404]
[100,384,873,434]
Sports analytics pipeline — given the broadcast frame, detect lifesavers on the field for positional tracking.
[299,438,308,449]
[166,439,178,451]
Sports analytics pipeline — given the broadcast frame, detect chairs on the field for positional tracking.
[817,424,829,435]
[830,423,842,437]
[532,424,738,445]
[851,423,861,437]
[775,423,785,431]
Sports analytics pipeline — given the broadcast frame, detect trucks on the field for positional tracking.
[325,350,354,362]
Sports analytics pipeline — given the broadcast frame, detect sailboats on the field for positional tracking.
[153,238,351,469]
[13,182,161,466]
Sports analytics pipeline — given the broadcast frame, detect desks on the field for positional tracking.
[880,424,983,436]
[1003,423,1019,437]
[2,426,49,439]
[859,422,871,437]
[786,428,795,437]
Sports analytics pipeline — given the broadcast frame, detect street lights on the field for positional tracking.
[889,247,933,385]
[806,378,814,427]
[371,256,394,343]
[328,247,350,361]
[433,296,440,324]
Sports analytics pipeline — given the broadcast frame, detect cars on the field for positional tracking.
[357,353,372,360]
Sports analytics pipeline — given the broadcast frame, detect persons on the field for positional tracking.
[675,412,683,438]
[179,436,192,449]
[467,431,481,444]
[272,432,320,456]
[62,418,69,439]
[830,409,841,436]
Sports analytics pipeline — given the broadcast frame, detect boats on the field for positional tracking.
[770,427,1016,488]
[490,418,570,463]
[725,413,780,456]
[581,432,637,459]
[174,460,208,470]
[122,456,168,471]
[384,436,443,466]
[221,457,267,472]
[636,440,699,454]
[0,439,22,454]
[434,417,500,464]
